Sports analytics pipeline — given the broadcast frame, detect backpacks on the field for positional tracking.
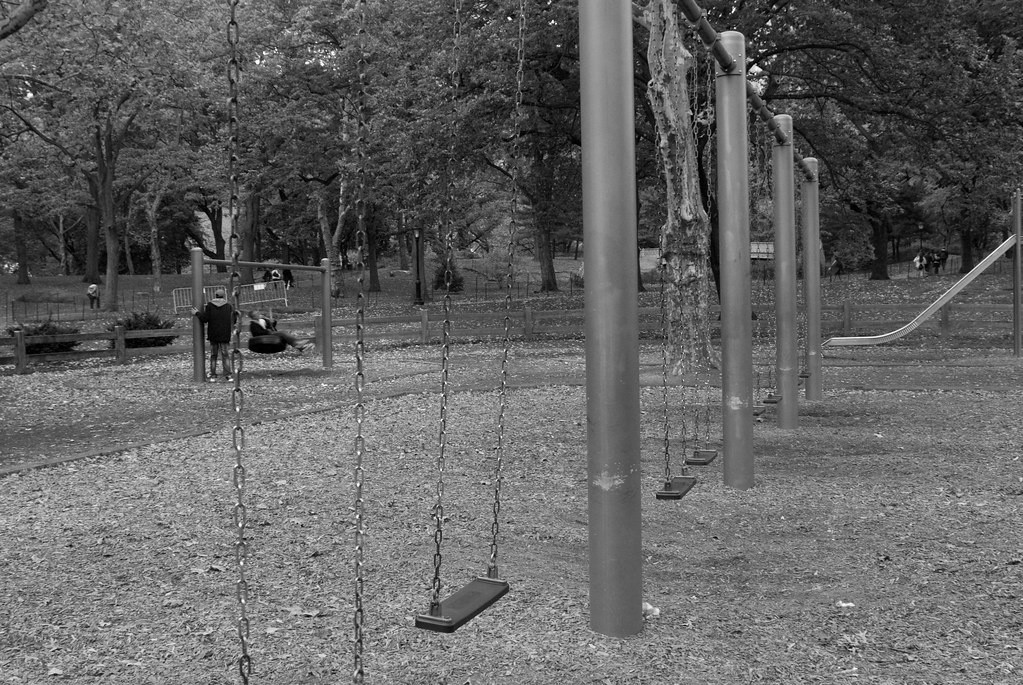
[87,287,96,299]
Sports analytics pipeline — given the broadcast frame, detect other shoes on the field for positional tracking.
[300,343,314,353]
[226,375,234,381]
[294,340,309,348]
[206,373,216,383]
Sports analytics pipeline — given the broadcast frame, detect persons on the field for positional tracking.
[191,290,237,382]
[88,280,100,310]
[829,256,844,283]
[263,269,293,287]
[913,248,948,278]
[249,311,313,351]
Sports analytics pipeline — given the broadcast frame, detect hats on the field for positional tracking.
[215,289,225,296]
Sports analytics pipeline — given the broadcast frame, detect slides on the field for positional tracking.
[820,234,1016,347]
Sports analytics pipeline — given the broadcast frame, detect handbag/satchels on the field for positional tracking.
[273,271,279,277]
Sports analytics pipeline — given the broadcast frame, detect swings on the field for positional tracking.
[653,0,813,502]
[248,272,287,354]
[410,1,527,634]
[224,0,366,685]
